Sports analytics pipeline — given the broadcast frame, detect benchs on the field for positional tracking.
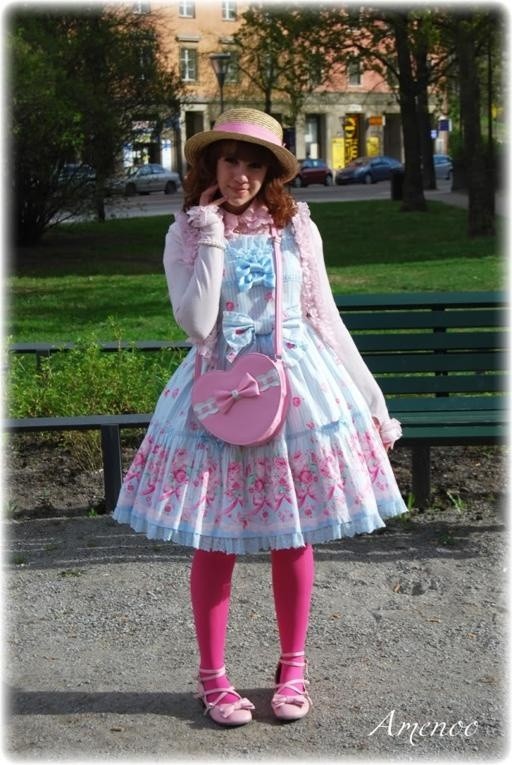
[326,288,508,515]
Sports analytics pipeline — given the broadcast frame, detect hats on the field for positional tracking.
[184,108,302,186]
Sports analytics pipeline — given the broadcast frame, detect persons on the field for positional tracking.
[108,108,411,726]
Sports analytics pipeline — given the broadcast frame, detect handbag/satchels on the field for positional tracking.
[190,353,287,447]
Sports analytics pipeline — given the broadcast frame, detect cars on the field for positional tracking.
[334,154,401,187]
[106,162,182,198]
[433,152,453,181]
[282,158,334,188]
[56,162,94,189]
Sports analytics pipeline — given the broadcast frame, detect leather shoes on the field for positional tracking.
[194,669,254,727]
[270,652,312,721]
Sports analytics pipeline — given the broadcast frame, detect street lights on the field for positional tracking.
[208,38,232,115]
